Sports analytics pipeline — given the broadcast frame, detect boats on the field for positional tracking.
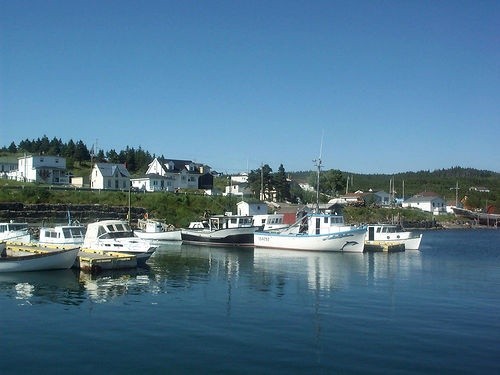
[0,241,81,274]
[364,224,423,251]
[132,223,183,241]
[1,220,158,266]
[180,215,265,246]
[254,213,368,254]
